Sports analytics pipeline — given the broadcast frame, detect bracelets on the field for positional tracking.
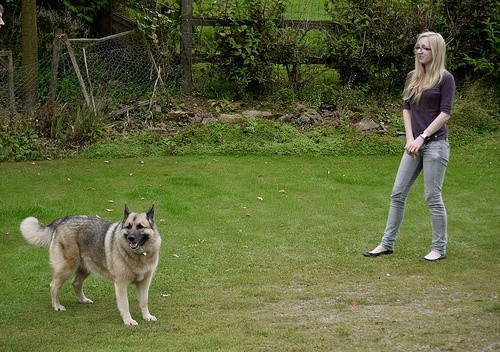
[419,129,431,141]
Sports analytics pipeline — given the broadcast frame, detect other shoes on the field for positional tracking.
[363,250,394,257]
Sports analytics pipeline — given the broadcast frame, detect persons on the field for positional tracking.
[362,32,455,262]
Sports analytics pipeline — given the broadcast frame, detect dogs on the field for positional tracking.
[19,202,162,327]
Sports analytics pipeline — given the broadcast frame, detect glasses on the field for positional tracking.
[414,45,433,53]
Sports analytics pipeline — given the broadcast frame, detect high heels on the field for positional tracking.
[419,255,446,261]
